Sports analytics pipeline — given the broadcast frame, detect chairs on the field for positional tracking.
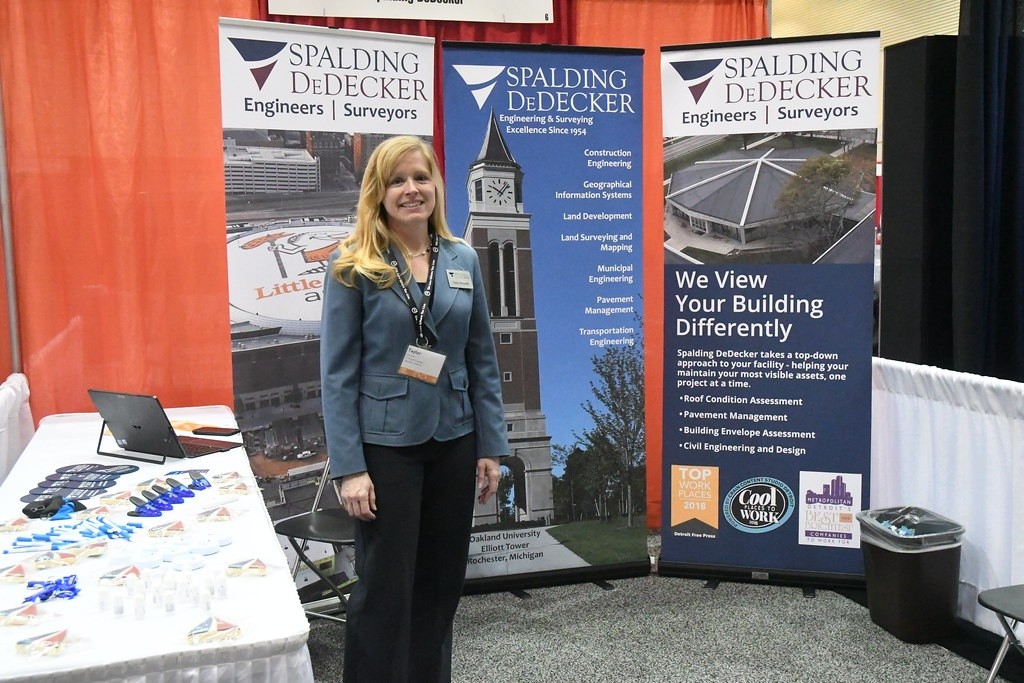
[0,373,36,485]
[274,457,355,623]
[978,584,1024,683]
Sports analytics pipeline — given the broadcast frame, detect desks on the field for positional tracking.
[0,406,313,683]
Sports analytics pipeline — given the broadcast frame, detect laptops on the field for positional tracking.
[87,389,245,459]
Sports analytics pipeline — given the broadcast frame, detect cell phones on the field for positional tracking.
[192,426,241,436]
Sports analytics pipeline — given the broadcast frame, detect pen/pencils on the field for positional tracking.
[3,514,146,553]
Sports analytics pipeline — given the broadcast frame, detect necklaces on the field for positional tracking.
[407,232,434,259]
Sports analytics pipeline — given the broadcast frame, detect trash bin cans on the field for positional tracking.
[855,508,966,648]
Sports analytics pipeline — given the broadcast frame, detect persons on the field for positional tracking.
[319,136,509,683]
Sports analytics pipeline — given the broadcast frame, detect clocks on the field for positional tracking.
[484,177,515,207]
